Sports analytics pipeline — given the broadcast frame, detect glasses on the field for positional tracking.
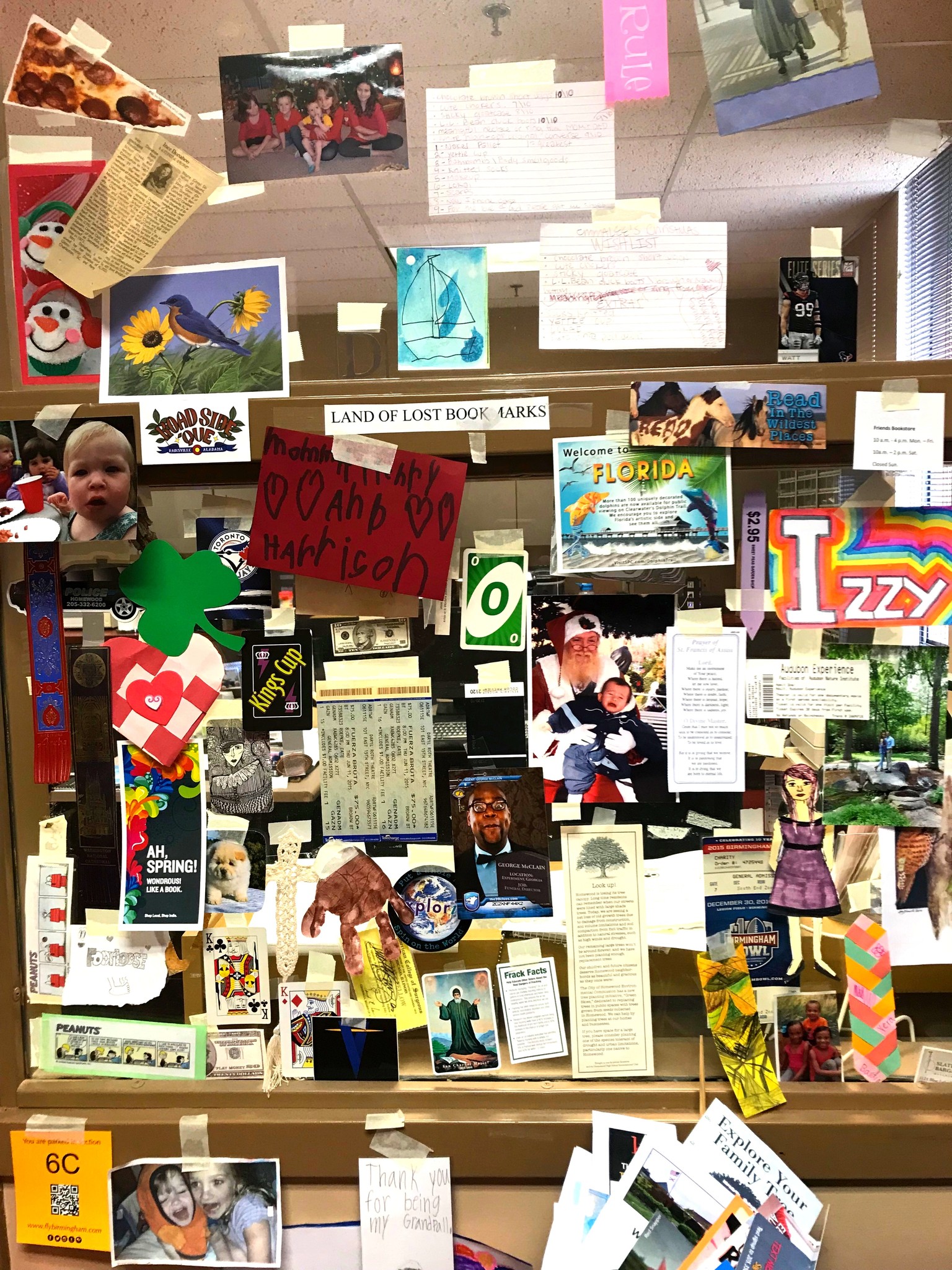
[468,799,509,814]
[569,641,598,652]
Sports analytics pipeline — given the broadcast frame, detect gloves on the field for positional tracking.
[604,728,636,754]
[569,724,596,746]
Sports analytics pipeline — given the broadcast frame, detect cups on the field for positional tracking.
[14,475,44,514]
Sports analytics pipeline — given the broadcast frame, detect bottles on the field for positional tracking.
[279,590,293,608]
[575,582,595,595]
[11,458,24,484]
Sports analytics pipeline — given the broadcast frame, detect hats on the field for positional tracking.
[565,614,602,644]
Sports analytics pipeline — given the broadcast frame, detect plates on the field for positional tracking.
[0,500,25,522]
[0,517,61,543]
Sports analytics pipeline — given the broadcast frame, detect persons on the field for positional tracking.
[529,609,668,803]
[0,421,138,543]
[875,730,895,774]
[738,0,849,74]
[135,1164,277,1264]
[453,781,551,904]
[232,78,403,173]
[780,271,822,349]
[778,999,842,1082]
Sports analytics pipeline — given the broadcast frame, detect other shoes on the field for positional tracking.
[875,766,879,768]
[885,770,892,773]
[884,768,888,770]
[880,768,883,771]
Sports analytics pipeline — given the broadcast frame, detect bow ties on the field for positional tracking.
[477,852,509,866]
